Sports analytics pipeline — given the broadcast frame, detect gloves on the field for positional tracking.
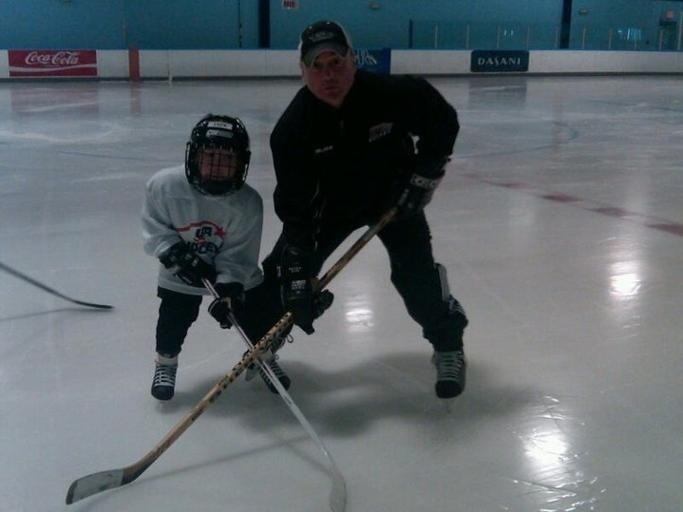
[176,251,217,288]
[209,283,244,330]
[376,172,445,224]
[280,242,335,329]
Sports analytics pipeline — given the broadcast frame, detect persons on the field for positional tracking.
[139,114,291,401]
[250,19,471,399]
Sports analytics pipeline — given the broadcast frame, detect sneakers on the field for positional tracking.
[261,357,292,393]
[431,346,467,400]
[152,361,177,400]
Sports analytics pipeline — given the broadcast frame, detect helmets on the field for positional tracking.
[182,111,252,198]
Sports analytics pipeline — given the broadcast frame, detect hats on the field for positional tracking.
[298,20,352,68]
